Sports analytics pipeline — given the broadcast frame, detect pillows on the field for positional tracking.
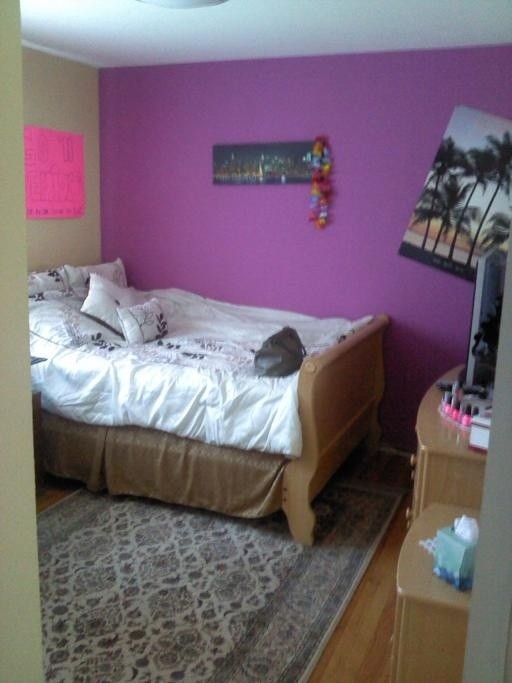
[28,257,168,344]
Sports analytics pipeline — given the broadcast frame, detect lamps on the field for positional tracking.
[136,0,226,10]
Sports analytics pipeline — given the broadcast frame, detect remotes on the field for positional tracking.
[436,379,490,399]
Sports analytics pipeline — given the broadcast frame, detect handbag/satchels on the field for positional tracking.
[254,327,307,378]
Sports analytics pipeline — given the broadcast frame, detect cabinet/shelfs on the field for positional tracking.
[387,503,481,683]
[405,363,487,531]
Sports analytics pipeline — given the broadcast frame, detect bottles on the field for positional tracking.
[442,381,478,426]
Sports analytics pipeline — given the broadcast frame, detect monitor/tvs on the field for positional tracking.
[459,245,506,388]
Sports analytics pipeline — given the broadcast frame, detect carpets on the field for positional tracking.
[37,446,417,683]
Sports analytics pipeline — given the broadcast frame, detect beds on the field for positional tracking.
[29,288,390,547]
